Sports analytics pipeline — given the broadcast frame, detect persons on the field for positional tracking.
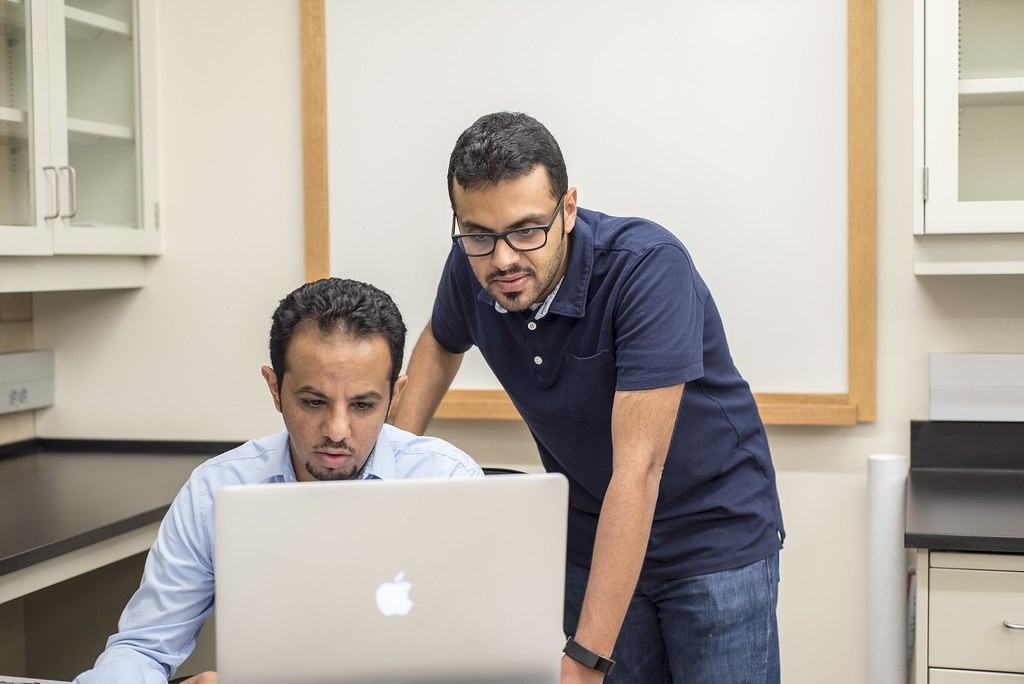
[71,277,486,684]
[391,110,790,684]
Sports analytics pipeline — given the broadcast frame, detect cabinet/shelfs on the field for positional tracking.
[913,548,1024,684]
[912,0,1024,277]
[0,0,159,292]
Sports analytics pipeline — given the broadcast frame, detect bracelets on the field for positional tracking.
[562,636,616,677]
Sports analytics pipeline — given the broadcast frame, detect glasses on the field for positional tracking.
[451,192,565,257]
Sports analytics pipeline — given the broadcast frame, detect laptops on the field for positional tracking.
[212,472,569,684]
